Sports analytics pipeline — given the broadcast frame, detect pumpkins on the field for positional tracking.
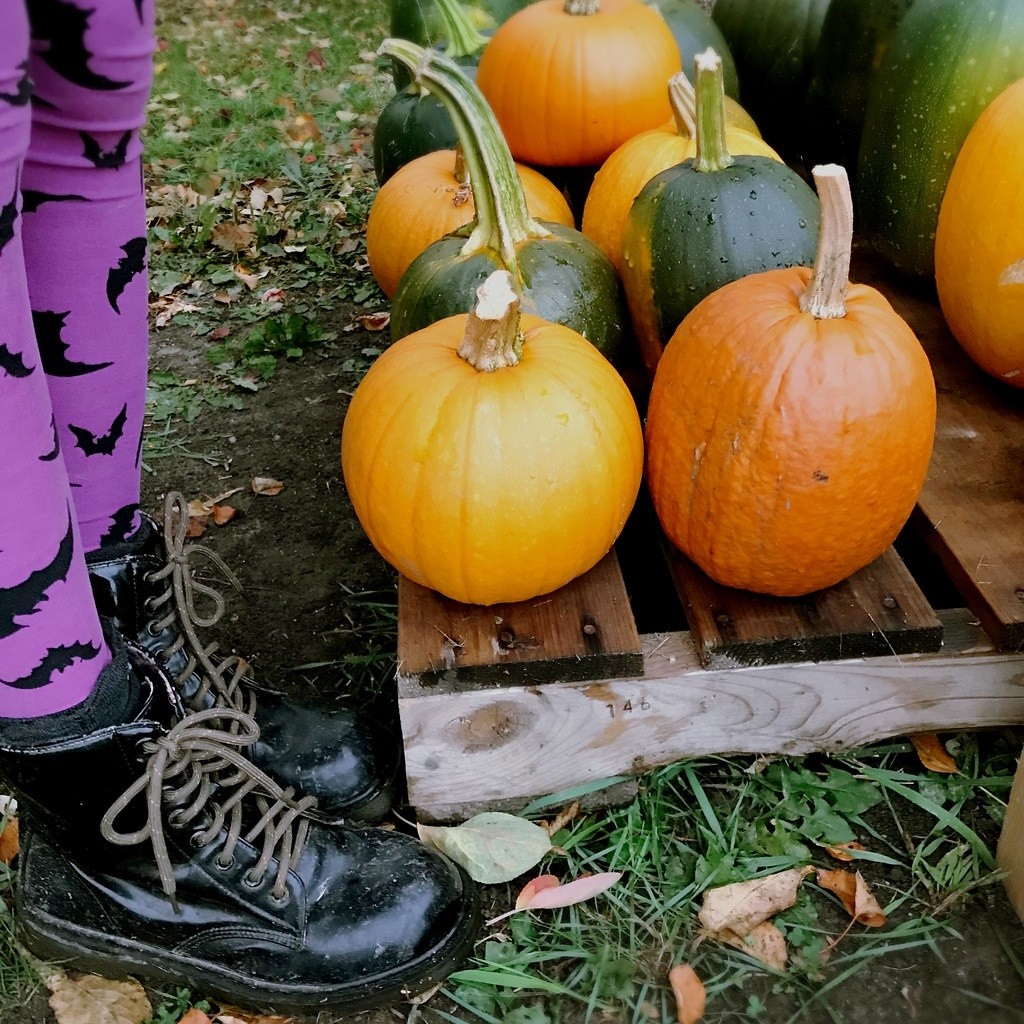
[343,0,1024,606]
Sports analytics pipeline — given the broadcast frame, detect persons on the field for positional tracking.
[0,0,478,1019]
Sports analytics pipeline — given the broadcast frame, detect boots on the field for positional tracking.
[84,489,402,824]
[0,674,483,1017]
